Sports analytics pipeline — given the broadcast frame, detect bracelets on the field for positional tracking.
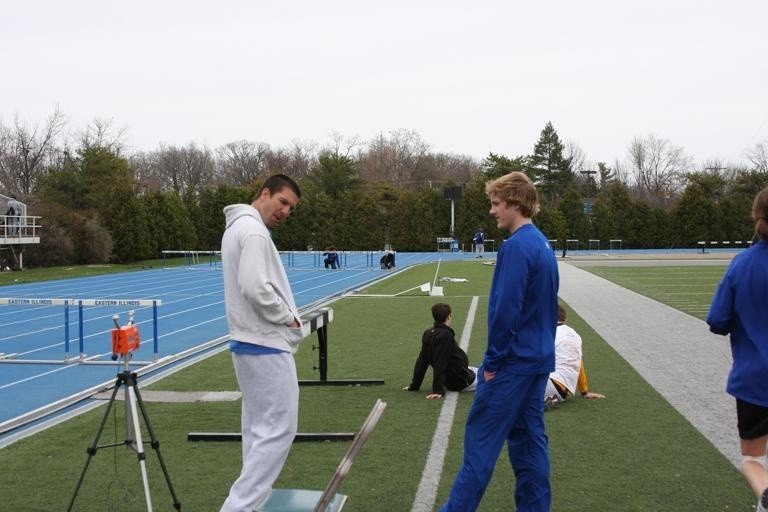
[580,391,587,397]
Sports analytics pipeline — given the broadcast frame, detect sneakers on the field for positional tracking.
[544,394,558,411]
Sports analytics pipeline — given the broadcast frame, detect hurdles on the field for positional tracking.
[549,240,558,254]
[183,304,386,441]
[610,239,622,251]
[276,250,395,272]
[587,239,601,252]
[471,239,495,255]
[436,236,454,252]
[0,297,172,370]
[697,241,753,254]
[566,239,579,252]
[163,251,223,271]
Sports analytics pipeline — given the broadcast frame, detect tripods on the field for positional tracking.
[67,365,183,512]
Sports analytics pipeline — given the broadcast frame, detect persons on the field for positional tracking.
[6,195,21,235]
[322,246,341,270]
[218,174,305,512]
[401,302,479,400]
[543,304,606,410]
[438,172,559,512]
[706,187,767,512]
[474,227,485,258]
[380,249,393,270]
